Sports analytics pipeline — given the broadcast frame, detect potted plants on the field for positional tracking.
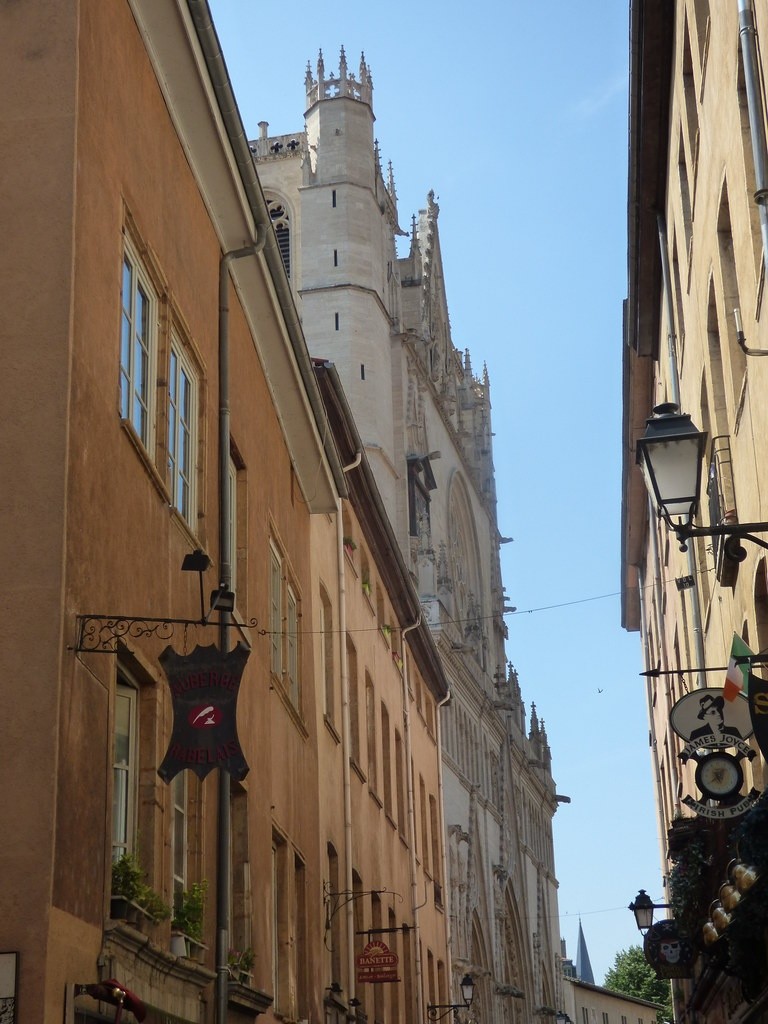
[110,853,171,930]
[171,878,209,959]
[362,581,373,596]
[382,625,392,639]
[344,536,357,558]
[228,946,258,982]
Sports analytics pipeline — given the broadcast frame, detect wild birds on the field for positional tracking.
[598,688,603,693]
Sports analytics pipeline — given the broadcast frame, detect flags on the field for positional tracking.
[723,631,755,702]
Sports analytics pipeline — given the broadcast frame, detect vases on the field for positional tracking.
[392,652,400,662]
[397,659,403,670]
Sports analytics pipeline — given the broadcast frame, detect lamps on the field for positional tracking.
[554,1011,571,1024]
[634,403,768,562]
[349,998,361,1007]
[325,983,344,993]
[180,549,210,624]
[206,582,235,624]
[628,889,674,936]
[427,973,475,1022]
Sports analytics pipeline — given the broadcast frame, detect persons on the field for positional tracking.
[689,694,743,746]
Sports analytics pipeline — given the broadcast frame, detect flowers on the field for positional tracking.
[227,948,240,964]
